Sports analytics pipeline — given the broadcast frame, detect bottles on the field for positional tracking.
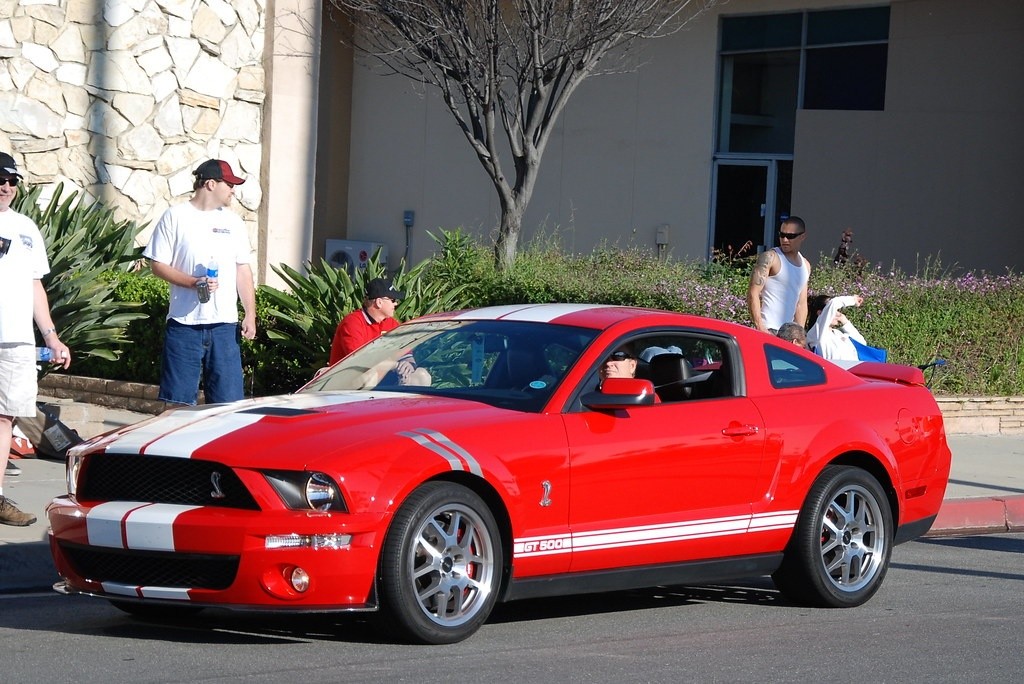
[36,347,67,361]
[206,256,219,283]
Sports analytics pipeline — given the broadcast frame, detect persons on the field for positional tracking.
[777,322,806,347]
[142,159,256,405]
[746,217,811,336]
[805,296,867,361]
[0,151,71,527]
[595,345,662,403]
[329,278,432,386]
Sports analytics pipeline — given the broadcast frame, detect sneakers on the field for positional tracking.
[0,494,37,526]
[4,460,21,475]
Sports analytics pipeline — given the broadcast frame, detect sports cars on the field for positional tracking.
[42,299,955,649]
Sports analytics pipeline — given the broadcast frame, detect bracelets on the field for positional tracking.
[41,328,56,337]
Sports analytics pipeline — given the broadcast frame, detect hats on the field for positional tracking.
[0,151,23,179]
[191,159,245,186]
[367,278,404,300]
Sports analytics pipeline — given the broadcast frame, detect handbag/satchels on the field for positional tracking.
[849,336,887,363]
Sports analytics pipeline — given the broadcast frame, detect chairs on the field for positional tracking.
[545,342,581,377]
[649,352,697,399]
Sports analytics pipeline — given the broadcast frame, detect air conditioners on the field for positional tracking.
[324,239,387,283]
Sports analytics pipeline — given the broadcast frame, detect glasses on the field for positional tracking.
[380,297,396,303]
[219,180,234,188]
[0,177,19,186]
[779,230,804,239]
[788,338,806,349]
[609,350,636,362]
